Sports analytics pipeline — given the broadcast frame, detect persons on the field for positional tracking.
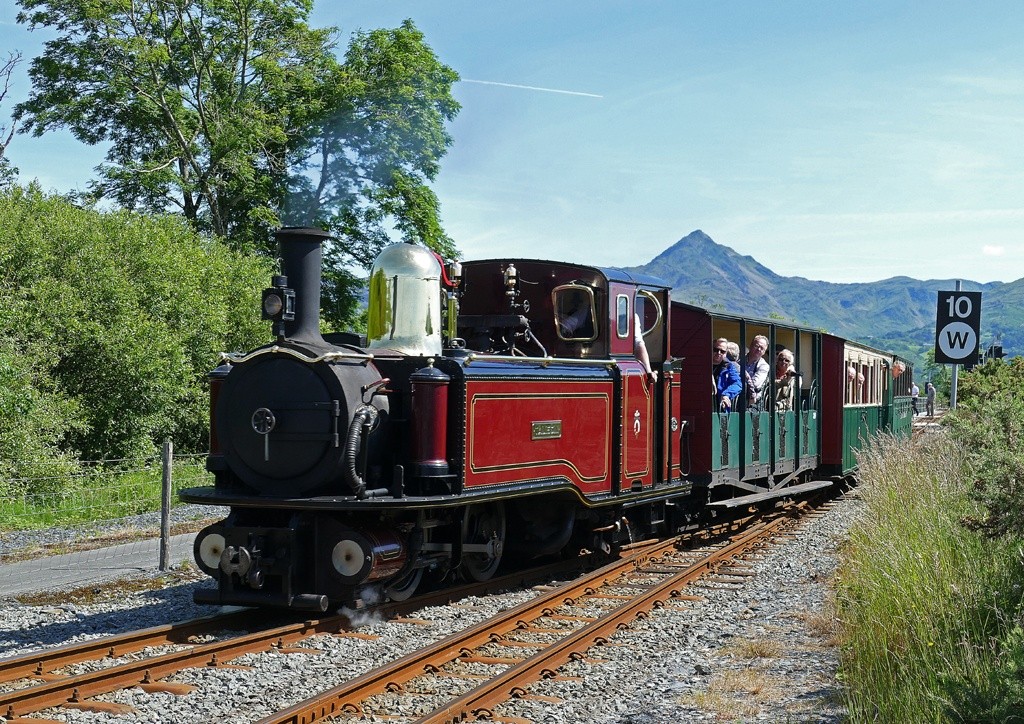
[926,383,936,416]
[713,334,803,412]
[560,290,590,336]
[848,366,865,389]
[634,312,658,383]
[893,361,905,380]
[911,382,919,416]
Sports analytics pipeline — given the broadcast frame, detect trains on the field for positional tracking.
[178,225,915,618]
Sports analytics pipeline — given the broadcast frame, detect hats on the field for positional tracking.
[776,344,785,350]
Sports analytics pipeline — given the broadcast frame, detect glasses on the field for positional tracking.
[776,352,779,355]
[713,347,727,355]
[780,358,790,362]
[753,344,766,351]
